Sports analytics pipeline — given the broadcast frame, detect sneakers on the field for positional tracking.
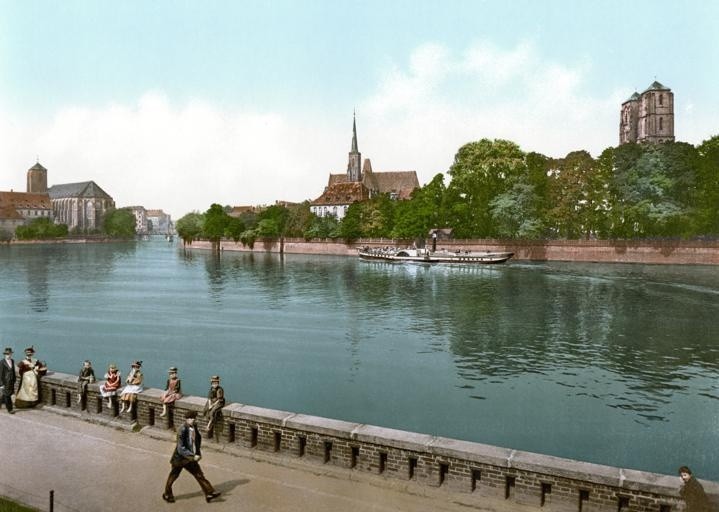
[206,492,221,501]
[9,410,15,413]
[163,493,175,502]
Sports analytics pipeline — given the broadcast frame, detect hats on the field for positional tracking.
[211,376,219,382]
[4,348,14,354]
[168,367,177,373]
[24,345,34,352]
[185,411,197,418]
[130,360,141,366]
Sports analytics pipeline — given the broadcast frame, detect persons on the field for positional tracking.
[14,345,47,409]
[161,412,221,504]
[158,367,184,417]
[676,464,709,512]
[76,360,96,405]
[0,347,17,415]
[200,376,227,432]
[119,361,143,413]
[100,362,121,409]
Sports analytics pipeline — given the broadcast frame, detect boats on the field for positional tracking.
[354,242,513,267]
